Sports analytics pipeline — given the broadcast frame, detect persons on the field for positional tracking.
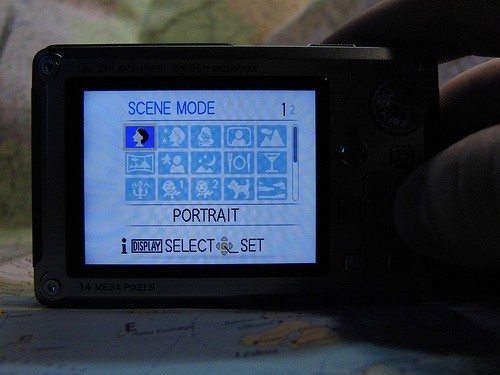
[331,50,500,356]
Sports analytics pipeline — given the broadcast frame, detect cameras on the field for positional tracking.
[26,40,442,309]
